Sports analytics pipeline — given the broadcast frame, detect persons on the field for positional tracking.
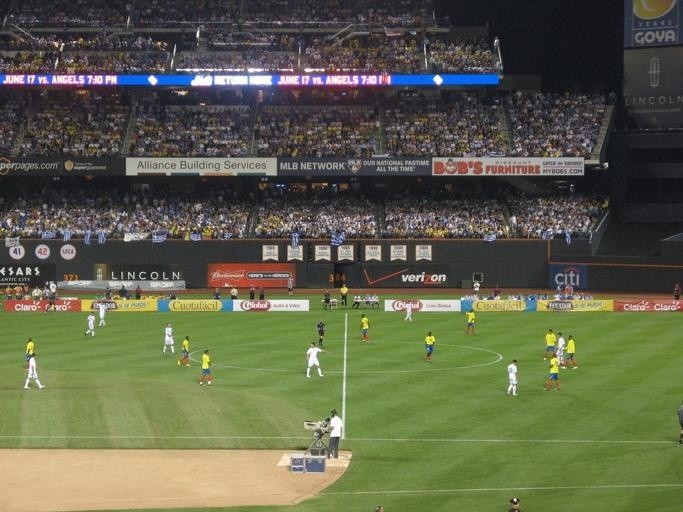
[325,284,379,310]
[326,409,344,460]
[85,301,108,337]
[673,284,681,300]
[676,400,683,445]
[507,359,518,396]
[305,343,330,378]
[287,275,294,295]
[23,338,45,389]
[542,328,578,392]
[213,284,264,299]
[404,300,413,322]
[316,319,327,346]
[507,498,525,512]
[162,323,212,387]
[425,331,436,361]
[105,285,176,300]
[359,313,370,343]
[5,281,58,314]
[0,0,617,240]
[461,308,475,335]
[473,279,586,300]
[374,506,384,512]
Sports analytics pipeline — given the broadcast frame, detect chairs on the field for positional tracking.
[322,295,379,310]
